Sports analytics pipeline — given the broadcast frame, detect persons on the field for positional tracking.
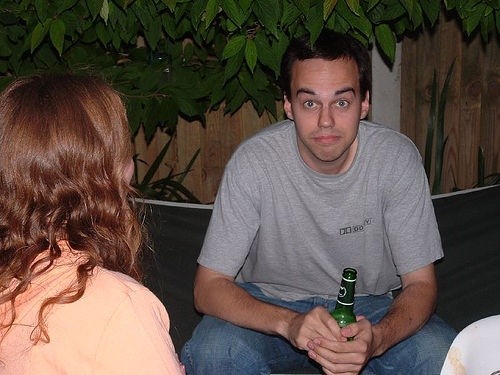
[0,70,186,375]
[180,27,459,375]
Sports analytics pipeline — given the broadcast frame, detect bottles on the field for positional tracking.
[330,268,360,342]
